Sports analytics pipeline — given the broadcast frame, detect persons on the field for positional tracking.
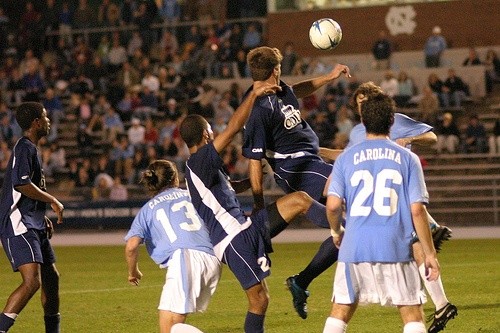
[322,93,439,333]
[241,46,351,320]
[125,159,222,333]
[0,102,64,333]
[180,84,346,333]
[317,81,452,256]
[0,1,500,204]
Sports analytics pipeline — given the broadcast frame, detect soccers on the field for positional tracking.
[309,18,343,51]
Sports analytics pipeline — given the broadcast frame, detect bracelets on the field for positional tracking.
[331,226,345,237]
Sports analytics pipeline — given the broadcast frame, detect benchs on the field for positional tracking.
[0,0,500,230]
